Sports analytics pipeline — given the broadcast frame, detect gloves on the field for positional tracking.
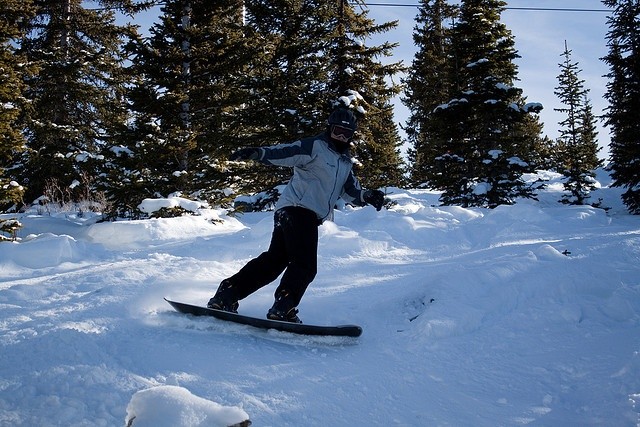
[363,189,385,211]
[228,147,261,164]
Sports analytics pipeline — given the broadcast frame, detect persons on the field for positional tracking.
[208,109,387,324]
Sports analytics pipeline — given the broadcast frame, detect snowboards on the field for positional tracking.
[164,297,362,337]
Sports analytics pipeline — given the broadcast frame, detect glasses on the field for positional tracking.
[332,125,355,138]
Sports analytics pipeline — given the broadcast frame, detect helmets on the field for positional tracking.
[328,108,359,131]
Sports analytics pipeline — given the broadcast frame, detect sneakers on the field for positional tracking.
[207,296,239,313]
[268,304,302,322]
